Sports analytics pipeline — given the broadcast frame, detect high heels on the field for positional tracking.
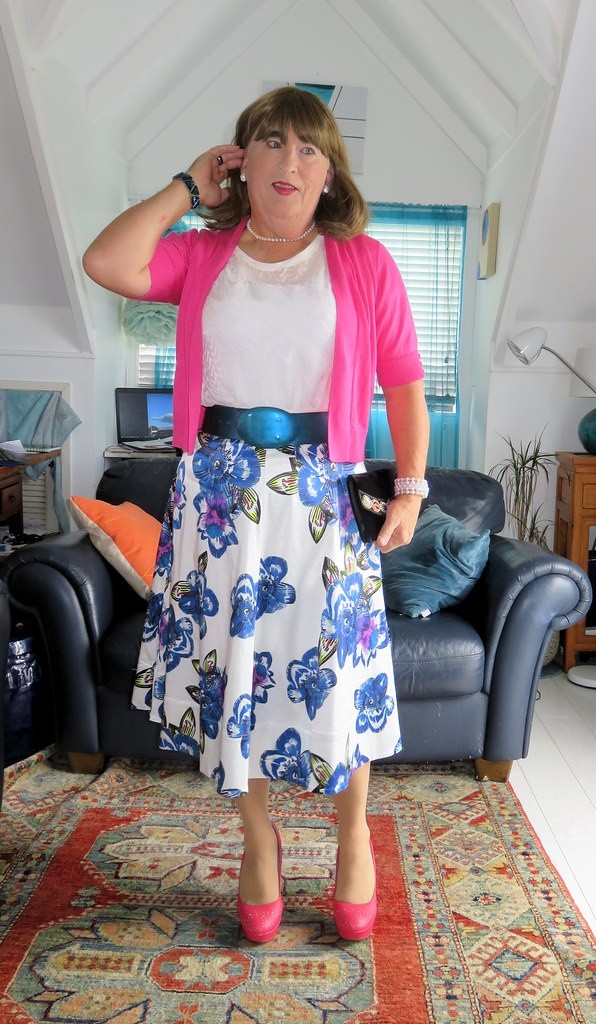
[331,836,378,941]
[237,820,284,943]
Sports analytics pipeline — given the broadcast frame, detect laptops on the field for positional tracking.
[115,388,177,452]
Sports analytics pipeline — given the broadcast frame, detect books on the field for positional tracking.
[21,443,60,453]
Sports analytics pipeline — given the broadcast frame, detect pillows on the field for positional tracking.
[62,493,165,597]
[376,504,493,621]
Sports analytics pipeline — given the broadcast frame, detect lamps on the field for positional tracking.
[506,325,596,397]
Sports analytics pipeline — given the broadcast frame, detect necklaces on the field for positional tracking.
[245,217,319,242]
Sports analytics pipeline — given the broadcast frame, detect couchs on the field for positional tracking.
[0,452,593,781]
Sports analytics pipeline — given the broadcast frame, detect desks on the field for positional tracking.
[549,450,595,678]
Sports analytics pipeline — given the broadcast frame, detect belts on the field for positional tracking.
[201,404,329,449]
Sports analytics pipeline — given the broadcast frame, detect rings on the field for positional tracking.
[216,154,224,166]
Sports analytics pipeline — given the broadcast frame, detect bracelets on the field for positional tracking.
[392,476,429,499]
[171,172,200,209]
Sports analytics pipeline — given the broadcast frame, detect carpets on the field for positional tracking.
[0,749,596,1024]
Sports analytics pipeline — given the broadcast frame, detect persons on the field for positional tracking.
[83,88,429,949]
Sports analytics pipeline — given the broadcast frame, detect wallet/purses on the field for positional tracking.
[347,468,396,544]
